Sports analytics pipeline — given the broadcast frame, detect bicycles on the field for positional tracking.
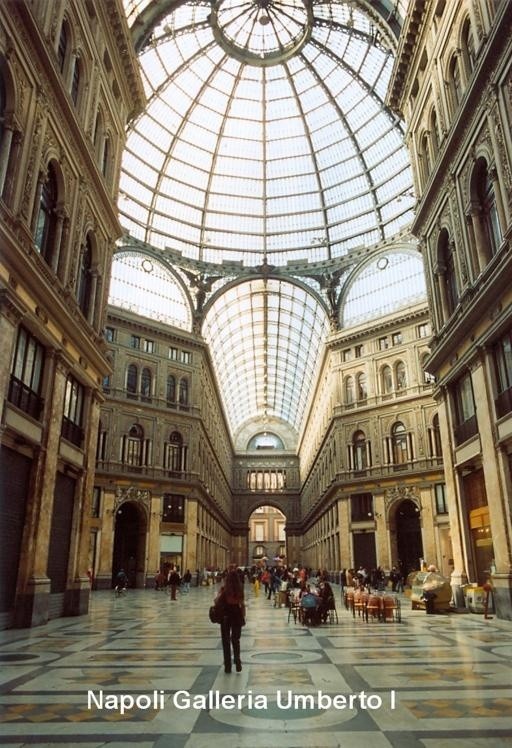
[113,584,129,598]
[164,577,190,596]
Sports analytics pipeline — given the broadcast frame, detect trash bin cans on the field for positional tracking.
[466,587,494,614]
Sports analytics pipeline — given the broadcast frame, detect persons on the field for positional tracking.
[340,565,407,595]
[115,567,128,593]
[154,556,335,672]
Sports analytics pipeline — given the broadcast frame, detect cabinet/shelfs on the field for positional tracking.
[404,571,453,612]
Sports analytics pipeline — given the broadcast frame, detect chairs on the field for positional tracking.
[276,575,403,627]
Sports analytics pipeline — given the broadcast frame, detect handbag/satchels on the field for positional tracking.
[210,605,223,623]
[302,593,316,608]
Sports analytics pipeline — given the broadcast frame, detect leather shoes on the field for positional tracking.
[225,661,231,672]
[234,656,242,672]
